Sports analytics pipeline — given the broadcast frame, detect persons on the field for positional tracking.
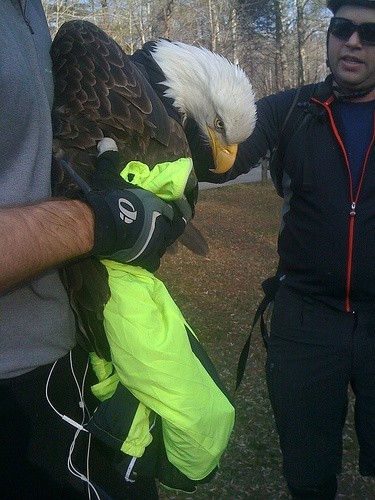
[185,0,375,500]
[0,0,231,500]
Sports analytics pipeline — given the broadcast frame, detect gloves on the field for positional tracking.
[76,137,188,272]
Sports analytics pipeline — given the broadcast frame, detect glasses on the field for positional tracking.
[329,17,375,44]
